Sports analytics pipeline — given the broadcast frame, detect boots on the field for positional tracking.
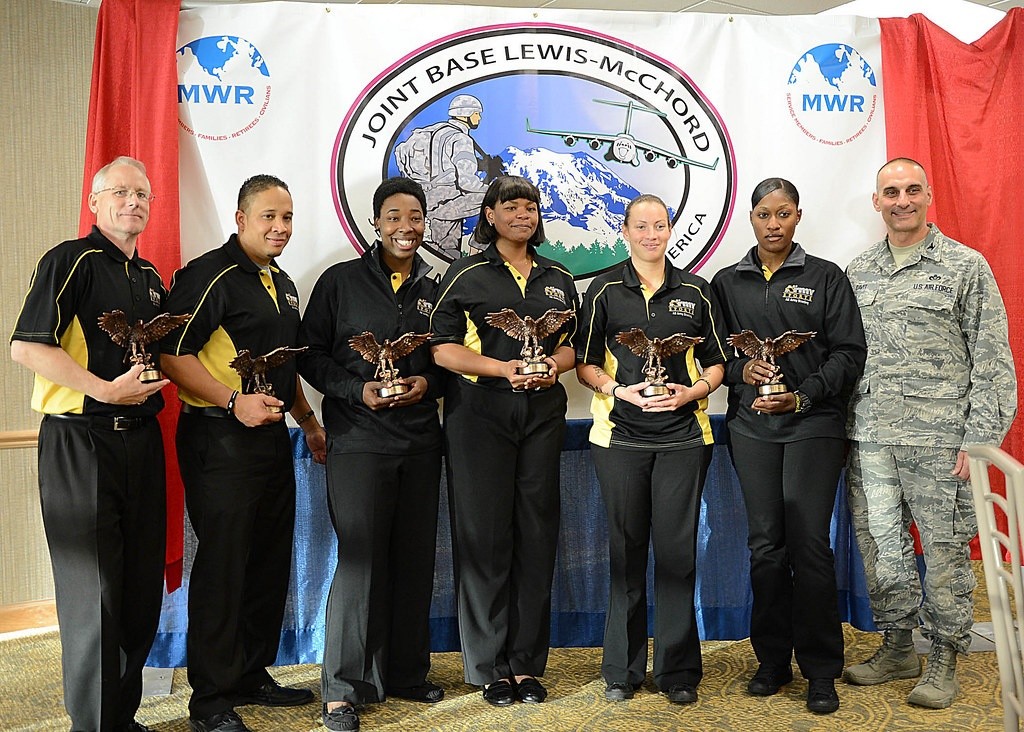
[908,637,961,709]
[844,630,922,685]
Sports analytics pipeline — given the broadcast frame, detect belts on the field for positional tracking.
[49,410,158,432]
[179,402,233,420]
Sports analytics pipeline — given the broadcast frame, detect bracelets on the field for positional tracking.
[795,394,800,413]
[550,355,556,363]
[296,409,315,424]
[226,390,239,414]
[612,383,626,401]
[693,379,711,400]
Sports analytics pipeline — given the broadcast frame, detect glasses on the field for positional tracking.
[95,185,156,203]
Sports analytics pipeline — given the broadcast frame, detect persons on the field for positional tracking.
[844,158,1018,708]
[10,157,168,732]
[581,194,733,705]
[711,175,867,715]
[297,176,444,732]
[162,175,313,732]
[425,178,579,706]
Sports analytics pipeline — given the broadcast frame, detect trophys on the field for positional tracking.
[230,346,309,413]
[615,328,705,397]
[349,331,434,399]
[99,310,192,381]
[726,329,816,395]
[483,308,576,377]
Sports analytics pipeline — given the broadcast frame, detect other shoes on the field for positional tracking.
[234,680,316,707]
[482,681,516,706]
[516,678,547,703]
[605,682,635,699]
[668,684,698,703]
[132,722,160,732]
[807,684,840,713]
[748,663,794,696]
[322,701,359,732]
[188,712,250,732]
[398,684,445,703]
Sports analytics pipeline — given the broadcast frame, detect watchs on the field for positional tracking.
[794,390,811,413]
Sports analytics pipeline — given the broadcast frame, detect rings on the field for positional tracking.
[749,364,755,372]
[756,411,761,415]
[535,386,540,390]
